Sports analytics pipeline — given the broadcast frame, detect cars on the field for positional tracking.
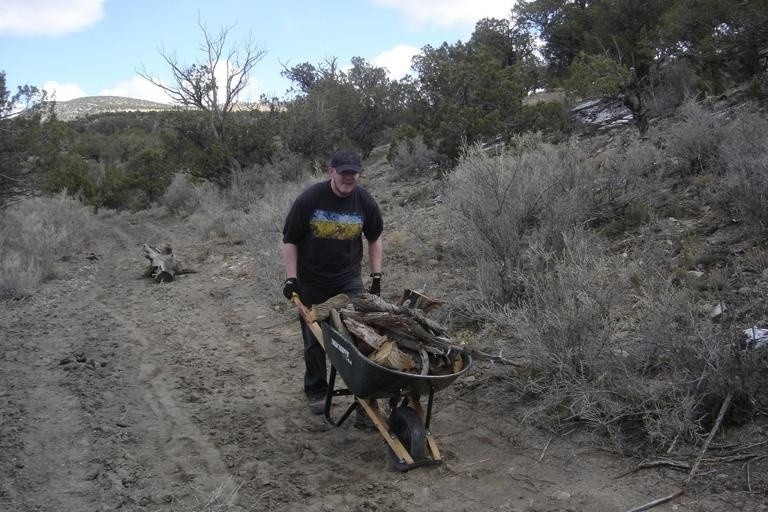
[561,99,610,139]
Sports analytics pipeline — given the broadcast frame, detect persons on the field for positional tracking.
[283,149,384,415]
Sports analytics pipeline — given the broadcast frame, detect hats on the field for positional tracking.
[329,148,364,175]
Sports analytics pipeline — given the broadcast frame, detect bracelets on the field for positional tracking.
[284,278,298,285]
[370,273,381,277]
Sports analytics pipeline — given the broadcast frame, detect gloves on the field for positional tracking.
[283,277,304,301]
[368,272,382,297]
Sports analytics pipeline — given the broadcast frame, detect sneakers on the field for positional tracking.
[309,394,328,414]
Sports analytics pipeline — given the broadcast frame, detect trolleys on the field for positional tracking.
[291,287,473,467]
